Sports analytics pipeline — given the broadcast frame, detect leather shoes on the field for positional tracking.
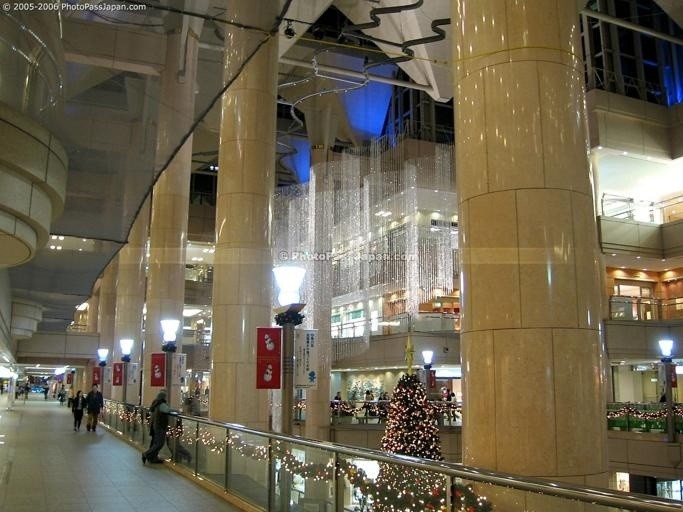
[149,459,163,464]
[142,452,147,463]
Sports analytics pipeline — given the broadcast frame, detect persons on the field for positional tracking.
[71,390,85,432]
[334,392,342,405]
[148,388,168,464]
[446,393,459,421]
[351,389,390,401]
[42,383,49,400]
[445,388,452,421]
[14,384,19,400]
[57,384,66,406]
[67,384,73,408]
[24,382,30,400]
[138,391,171,465]
[165,437,192,463]
[85,383,103,433]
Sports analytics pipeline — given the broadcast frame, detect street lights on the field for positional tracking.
[422,350,433,401]
[658,339,675,443]
[272,265,307,512]
[119,339,134,435]
[159,319,180,463]
[97,348,108,422]
[70,367,75,397]
